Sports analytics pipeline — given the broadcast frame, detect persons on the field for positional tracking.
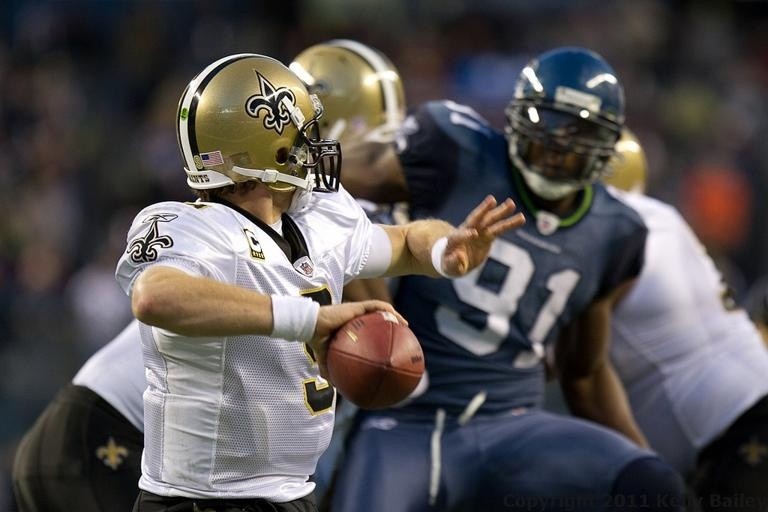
[114,52,527,511]
[546,122,767,508]
[309,45,704,511]
[11,37,420,511]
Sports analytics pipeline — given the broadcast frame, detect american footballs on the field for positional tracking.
[326,310,426,410]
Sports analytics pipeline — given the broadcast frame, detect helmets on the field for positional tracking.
[175,50,339,194]
[601,125,648,192]
[285,38,421,164]
[502,47,626,203]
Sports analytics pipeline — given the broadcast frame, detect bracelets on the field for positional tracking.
[270,291,320,342]
[432,237,453,279]
